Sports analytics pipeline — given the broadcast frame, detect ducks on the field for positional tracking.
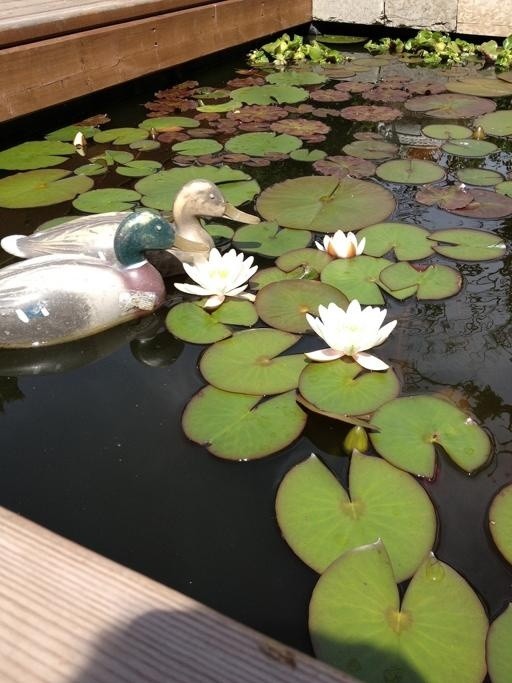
[0,205,210,352]
[0,178,262,280]
